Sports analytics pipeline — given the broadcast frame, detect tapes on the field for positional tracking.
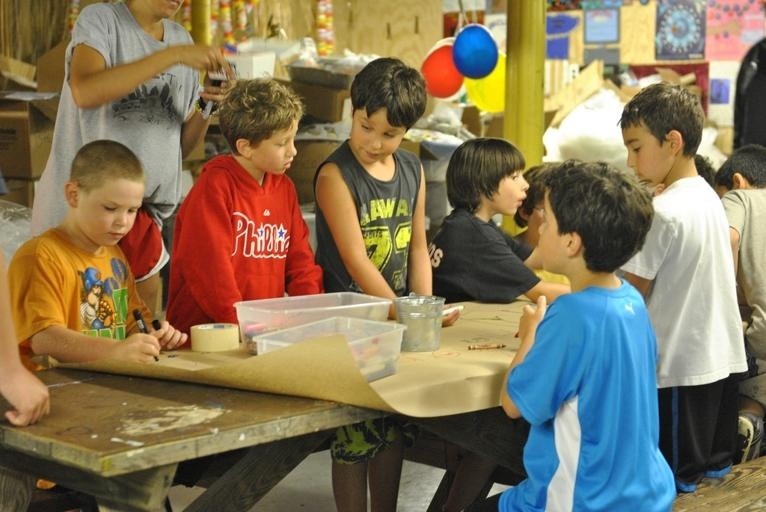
[191,323,240,352]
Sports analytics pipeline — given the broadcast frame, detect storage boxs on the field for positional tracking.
[280,78,352,124]
[0,42,69,210]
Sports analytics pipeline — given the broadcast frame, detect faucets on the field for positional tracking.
[263,13,288,42]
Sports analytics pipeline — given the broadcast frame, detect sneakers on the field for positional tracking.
[735,410,762,465]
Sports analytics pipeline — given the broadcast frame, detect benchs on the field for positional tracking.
[671,456,766,511]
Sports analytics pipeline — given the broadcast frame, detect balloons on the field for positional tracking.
[421,24,505,112]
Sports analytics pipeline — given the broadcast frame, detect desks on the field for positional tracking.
[0,301,553,511]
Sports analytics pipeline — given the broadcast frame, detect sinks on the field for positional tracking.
[235,32,301,59]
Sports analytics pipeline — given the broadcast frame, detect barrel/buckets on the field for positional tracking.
[392,291,446,353]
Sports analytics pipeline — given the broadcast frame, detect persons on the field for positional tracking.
[695,38,766,466]
[315,58,433,512]
[166,78,325,352]
[613,81,748,493]
[0,140,188,427]
[32,1,236,319]
[429,138,676,512]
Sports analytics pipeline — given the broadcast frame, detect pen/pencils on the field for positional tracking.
[467,344,507,350]
[133,309,160,362]
[515,332,520,338]
